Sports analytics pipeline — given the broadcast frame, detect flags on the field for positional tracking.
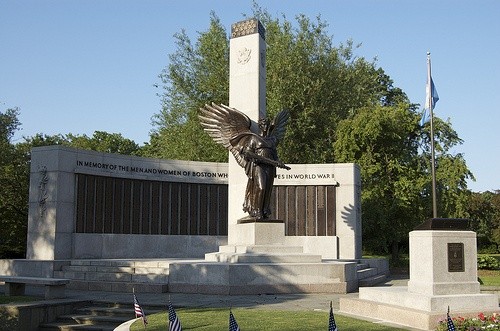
[447,313,456,331]
[328,301,338,331]
[168,300,182,331]
[229,312,240,331]
[420,60,439,126]
[133,293,148,327]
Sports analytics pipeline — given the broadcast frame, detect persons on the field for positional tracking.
[240,118,285,221]
